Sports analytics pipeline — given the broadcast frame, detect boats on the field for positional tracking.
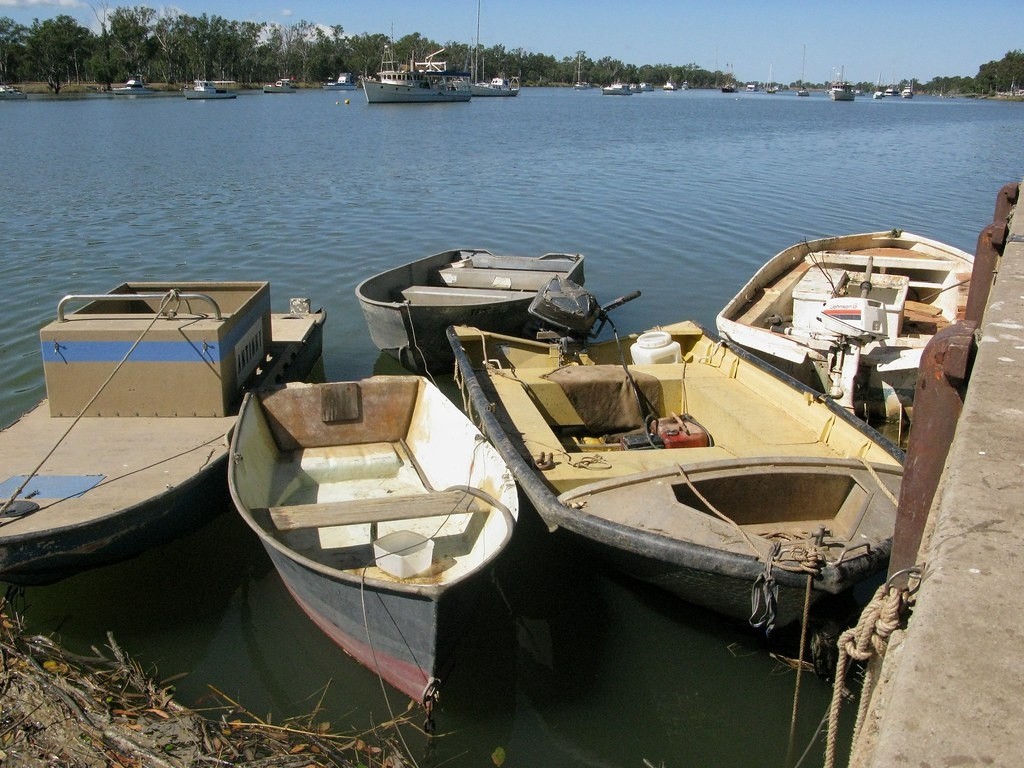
[356,246,586,375]
[470,76,520,97]
[183,79,236,99]
[228,376,521,710]
[1,280,328,589]
[571,67,914,103]
[112,80,152,94]
[322,77,357,91]
[445,318,907,634]
[361,43,471,103]
[0,84,27,99]
[716,228,977,446]
[263,79,296,94]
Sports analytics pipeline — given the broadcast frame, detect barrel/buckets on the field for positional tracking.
[629,331,682,364]
